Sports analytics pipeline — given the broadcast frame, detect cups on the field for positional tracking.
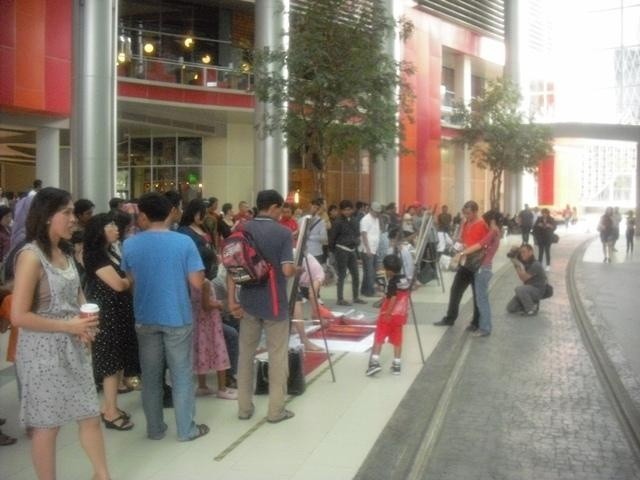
[80,302,101,331]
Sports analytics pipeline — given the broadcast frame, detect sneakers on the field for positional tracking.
[435,319,489,339]
[366,358,382,377]
[216,389,236,400]
[389,360,402,375]
[196,386,216,396]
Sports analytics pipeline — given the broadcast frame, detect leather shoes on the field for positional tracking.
[196,424,209,436]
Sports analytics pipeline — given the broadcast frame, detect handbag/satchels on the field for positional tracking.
[542,283,553,299]
[253,344,307,396]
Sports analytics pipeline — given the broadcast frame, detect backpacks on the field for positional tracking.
[221,222,279,317]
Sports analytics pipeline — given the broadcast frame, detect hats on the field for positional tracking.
[371,202,382,213]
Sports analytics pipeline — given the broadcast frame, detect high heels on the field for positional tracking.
[101,409,134,430]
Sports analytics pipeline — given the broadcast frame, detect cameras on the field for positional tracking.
[507,248,520,258]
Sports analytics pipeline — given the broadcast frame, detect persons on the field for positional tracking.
[366,255,410,375]
[326,200,557,307]
[562,203,577,227]
[625,209,635,254]
[507,244,548,316]
[598,206,623,265]
[0,180,330,442]
[430,201,503,336]
[9,186,113,480]
